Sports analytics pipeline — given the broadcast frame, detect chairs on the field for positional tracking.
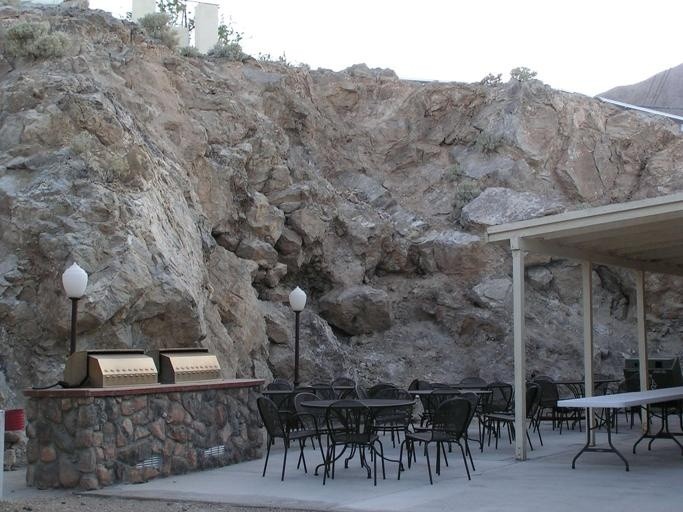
[615,373,653,434]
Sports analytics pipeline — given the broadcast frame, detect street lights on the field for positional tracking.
[288,286,306,388]
[59,263,88,354]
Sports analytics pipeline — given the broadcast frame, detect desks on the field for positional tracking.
[551,379,622,432]
[556,386,683,471]
